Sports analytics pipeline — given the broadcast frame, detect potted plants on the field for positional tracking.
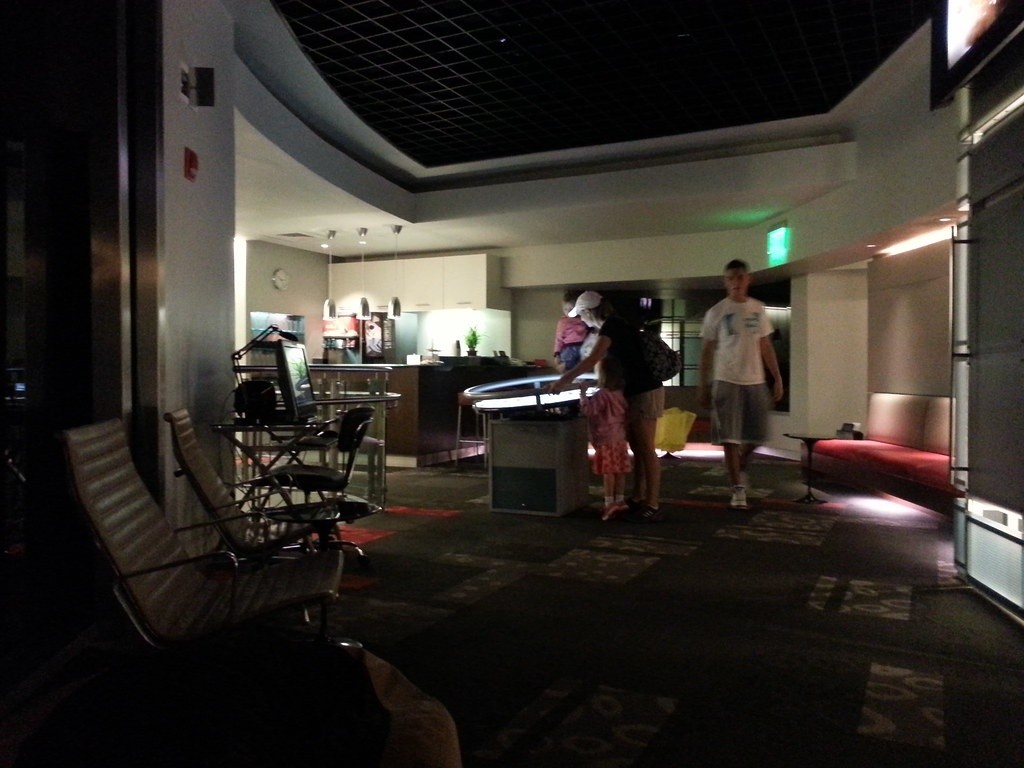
[467,332,478,356]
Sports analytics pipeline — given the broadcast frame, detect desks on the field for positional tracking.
[782,432,841,506]
[308,363,553,468]
[265,490,384,561]
[209,418,340,553]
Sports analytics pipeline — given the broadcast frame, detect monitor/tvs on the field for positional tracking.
[275,339,318,420]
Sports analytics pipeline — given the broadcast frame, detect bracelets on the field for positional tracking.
[702,384,712,389]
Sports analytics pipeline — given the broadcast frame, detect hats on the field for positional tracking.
[567,290,603,318]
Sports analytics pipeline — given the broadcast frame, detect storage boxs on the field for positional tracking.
[406,354,440,364]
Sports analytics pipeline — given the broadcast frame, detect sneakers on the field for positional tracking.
[741,471,752,498]
[730,483,748,510]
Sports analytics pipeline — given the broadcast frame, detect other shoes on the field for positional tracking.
[601,500,629,521]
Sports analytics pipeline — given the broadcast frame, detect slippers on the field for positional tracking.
[634,502,666,523]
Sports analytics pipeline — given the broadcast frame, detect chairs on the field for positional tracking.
[231,407,376,565]
[52,417,344,655]
[800,392,966,516]
[162,407,321,560]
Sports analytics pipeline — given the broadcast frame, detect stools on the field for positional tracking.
[454,393,493,471]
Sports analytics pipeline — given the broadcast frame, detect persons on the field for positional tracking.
[696,259,783,506]
[543,289,664,523]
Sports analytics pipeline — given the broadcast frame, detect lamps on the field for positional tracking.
[355,228,372,320]
[232,325,299,387]
[387,224,403,319]
[324,230,338,320]
[181,67,215,107]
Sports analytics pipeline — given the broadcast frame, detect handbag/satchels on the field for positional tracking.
[639,329,682,383]
